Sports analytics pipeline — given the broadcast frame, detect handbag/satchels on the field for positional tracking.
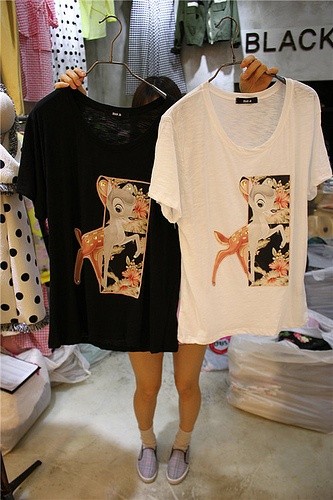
[200,241,333,434]
[1,343,111,457]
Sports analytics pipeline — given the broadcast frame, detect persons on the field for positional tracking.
[52,53,281,484]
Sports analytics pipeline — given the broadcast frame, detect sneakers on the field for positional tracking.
[166,445,190,484]
[137,444,158,482]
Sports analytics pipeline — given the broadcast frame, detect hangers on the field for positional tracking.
[207,16,287,86]
[70,15,167,99]
